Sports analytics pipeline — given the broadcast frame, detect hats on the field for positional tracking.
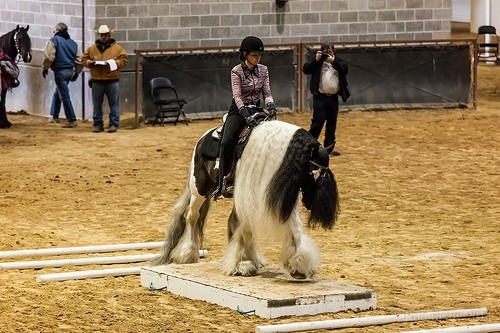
[98,24,110,34]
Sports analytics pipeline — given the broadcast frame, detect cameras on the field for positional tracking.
[320,52,330,61]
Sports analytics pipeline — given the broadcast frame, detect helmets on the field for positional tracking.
[239,36,265,53]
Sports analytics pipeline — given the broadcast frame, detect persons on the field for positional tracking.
[82,25,128,132]
[303,41,350,155]
[42,22,83,127]
[213,36,277,201]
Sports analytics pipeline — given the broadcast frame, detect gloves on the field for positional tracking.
[245,116,258,127]
[42,69,49,78]
[71,72,78,82]
[268,107,276,118]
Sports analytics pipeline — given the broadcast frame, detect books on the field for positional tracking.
[94,58,117,71]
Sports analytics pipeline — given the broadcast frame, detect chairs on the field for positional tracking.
[477,25,500,65]
[151,77,189,126]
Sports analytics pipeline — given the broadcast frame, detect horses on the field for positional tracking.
[0,22,36,130]
[152,119,341,282]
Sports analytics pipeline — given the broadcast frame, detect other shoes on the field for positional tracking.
[49,118,61,123]
[62,121,77,128]
[93,124,104,131]
[331,150,341,155]
[107,125,117,132]
[213,185,234,201]
